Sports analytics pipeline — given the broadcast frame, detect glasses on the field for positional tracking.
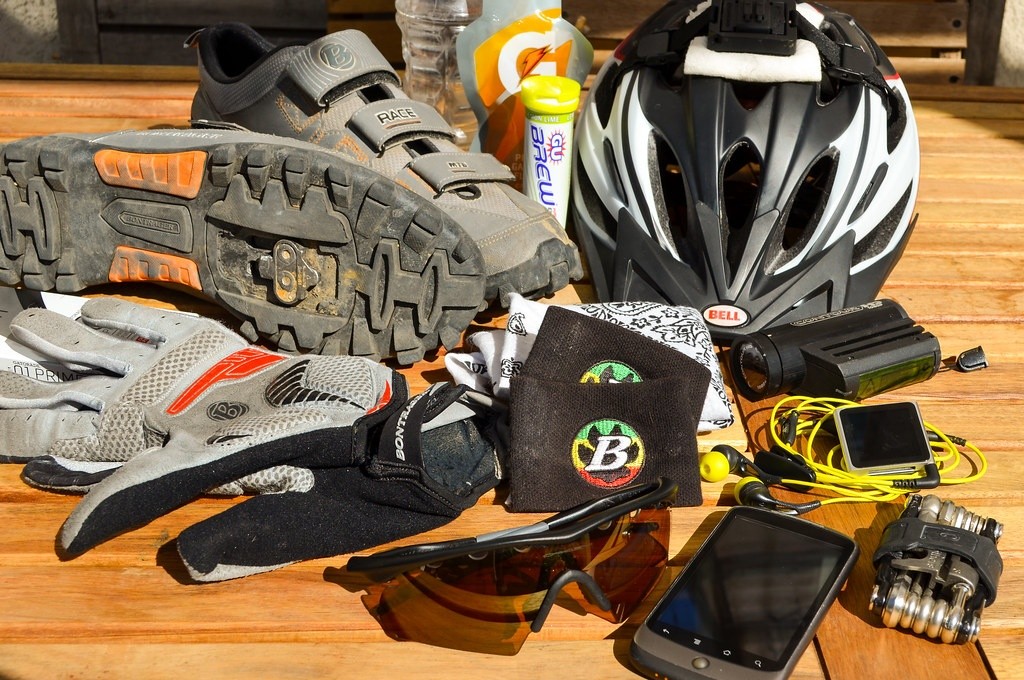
[346,476,678,657]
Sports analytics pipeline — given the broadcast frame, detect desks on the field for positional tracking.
[0,62,1024,680]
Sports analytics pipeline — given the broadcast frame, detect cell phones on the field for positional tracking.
[630,503,860,679]
[833,401,936,473]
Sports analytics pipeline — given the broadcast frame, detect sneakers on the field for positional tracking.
[0,118,487,366]
[187,21,586,313]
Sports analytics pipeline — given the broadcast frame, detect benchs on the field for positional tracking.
[57,0,969,86]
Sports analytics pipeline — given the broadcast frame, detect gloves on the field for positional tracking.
[1,297,507,583]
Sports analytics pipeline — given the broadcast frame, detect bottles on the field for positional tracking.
[456,0,594,194]
[521,77,580,231]
[394,0,484,154]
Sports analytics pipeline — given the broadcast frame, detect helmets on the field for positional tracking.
[567,0,920,340]
